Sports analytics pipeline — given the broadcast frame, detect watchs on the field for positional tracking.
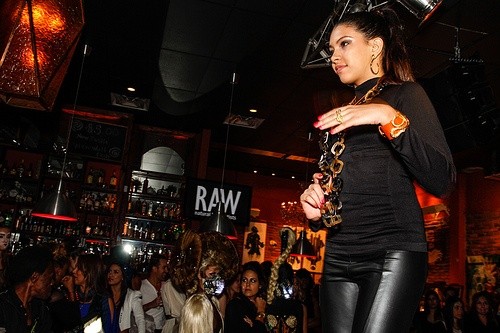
[257,312,266,319]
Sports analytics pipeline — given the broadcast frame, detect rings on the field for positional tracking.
[337,115,342,122]
[336,110,342,117]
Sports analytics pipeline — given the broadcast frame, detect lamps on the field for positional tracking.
[197,73,239,240]
[397,0,443,27]
[290,129,316,255]
[31,45,88,221]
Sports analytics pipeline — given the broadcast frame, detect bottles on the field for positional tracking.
[127,197,181,221]
[109,169,116,189]
[85,167,93,185]
[96,169,103,187]
[122,218,185,245]
[77,191,117,210]
[0,159,65,256]
[65,216,112,256]
[128,244,171,274]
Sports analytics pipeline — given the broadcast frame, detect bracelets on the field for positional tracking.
[379,116,409,140]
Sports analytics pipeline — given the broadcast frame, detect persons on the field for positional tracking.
[301,8,456,333]
[418,285,500,333]
[0,225,319,333]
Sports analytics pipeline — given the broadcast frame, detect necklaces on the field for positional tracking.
[318,83,387,228]
[76,288,93,304]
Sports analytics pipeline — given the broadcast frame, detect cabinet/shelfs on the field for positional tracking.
[113,168,188,272]
[0,170,121,263]
